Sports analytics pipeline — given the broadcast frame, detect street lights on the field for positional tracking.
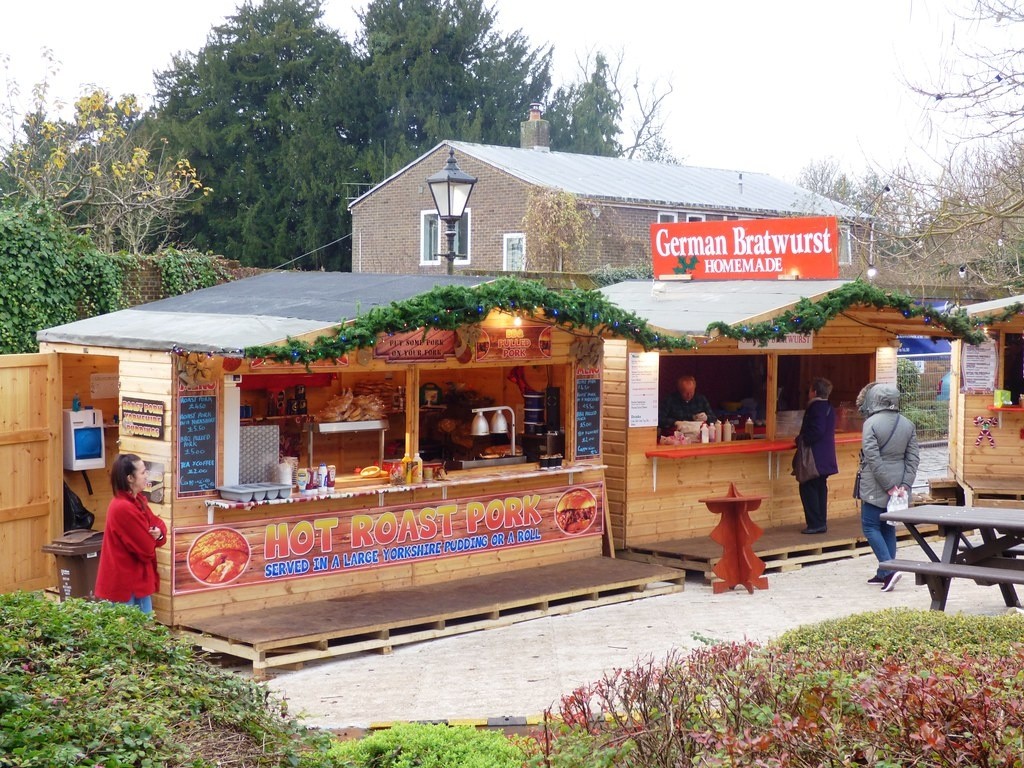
[426,147,478,277]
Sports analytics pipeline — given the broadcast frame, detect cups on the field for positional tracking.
[555,454,563,468]
[277,456,298,485]
[539,454,549,470]
[549,455,556,469]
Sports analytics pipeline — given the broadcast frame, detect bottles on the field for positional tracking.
[700,417,754,443]
[411,453,422,483]
[401,453,412,486]
[297,463,337,496]
[72,391,81,412]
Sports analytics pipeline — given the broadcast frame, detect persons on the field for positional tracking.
[93,452,167,619]
[658,374,718,438]
[793,378,840,535]
[856,381,920,592]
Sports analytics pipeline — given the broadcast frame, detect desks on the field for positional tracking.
[880,503,1024,562]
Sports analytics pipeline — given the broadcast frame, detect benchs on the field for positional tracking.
[875,560,1024,614]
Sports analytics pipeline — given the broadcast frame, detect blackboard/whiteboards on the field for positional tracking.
[179,394,217,495]
[574,378,601,458]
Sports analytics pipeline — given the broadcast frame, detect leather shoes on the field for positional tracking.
[801,525,827,534]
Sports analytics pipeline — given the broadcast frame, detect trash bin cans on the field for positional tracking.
[40,529,105,605]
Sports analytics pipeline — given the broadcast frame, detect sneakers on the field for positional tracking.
[881,570,902,592]
[866,575,884,585]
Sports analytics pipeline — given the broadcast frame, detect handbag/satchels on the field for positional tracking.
[886,488,909,526]
[790,438,820,483]
[852,470,860,500]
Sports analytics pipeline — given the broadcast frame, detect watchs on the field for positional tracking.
[157,534,164,541]
